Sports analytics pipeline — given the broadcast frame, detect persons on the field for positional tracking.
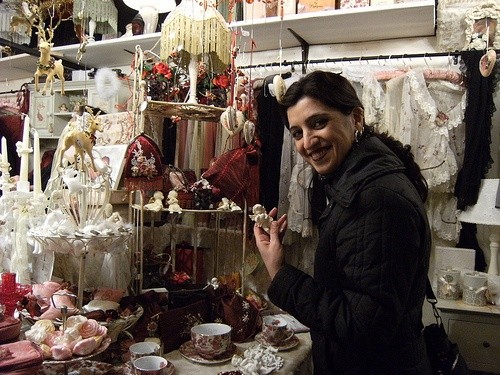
[132,14,145,35]
[248,203,283,235]
[254,71,431,375]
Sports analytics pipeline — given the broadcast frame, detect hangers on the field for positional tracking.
[235,49,469,87]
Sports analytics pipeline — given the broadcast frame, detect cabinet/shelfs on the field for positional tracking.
[27,79,137,140]
[435,294,500,375]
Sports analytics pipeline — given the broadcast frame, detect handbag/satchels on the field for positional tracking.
[211,284,264,343]
[424,320,470,375]
[117,285,217,354]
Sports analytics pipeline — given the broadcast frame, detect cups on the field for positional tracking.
[129,342,167,375]
[261,318,294,344]
[190,323,232,359]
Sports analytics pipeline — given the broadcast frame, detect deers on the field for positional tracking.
[59,106,103,173]
[33,23,66,96]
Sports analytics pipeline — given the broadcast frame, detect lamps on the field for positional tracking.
[453,176,500,297]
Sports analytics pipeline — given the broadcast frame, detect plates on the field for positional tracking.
[43,331,111,363]
[123,359,176,375]
[179,340,237,363]
[255,331,300,350]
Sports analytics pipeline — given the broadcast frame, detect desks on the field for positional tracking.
[161,332,314,375]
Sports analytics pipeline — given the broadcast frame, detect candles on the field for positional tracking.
[20,113,30,182]
[17,212,28,285]
[1,135,8,172]
[32,128,42,192]
